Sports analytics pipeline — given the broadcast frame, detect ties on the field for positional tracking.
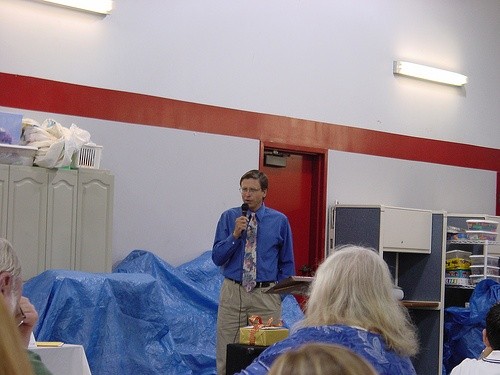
[241,212,258,293]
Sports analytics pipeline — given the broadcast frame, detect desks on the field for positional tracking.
[28,343,92,375]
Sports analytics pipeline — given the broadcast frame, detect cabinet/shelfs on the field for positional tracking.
[327,203,446,375]
[0,163,116,284]
[445,239,500,289]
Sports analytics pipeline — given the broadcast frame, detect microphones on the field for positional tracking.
[240,203,249,235]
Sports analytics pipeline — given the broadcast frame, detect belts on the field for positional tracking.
[228,278,275,288]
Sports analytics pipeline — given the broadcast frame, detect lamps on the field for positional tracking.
[45,0,113,15]
[393,60,469,88]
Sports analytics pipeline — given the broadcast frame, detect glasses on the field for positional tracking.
[13,300,27,326]
[239,186,261,195]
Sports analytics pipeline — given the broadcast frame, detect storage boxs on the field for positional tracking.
[71,144,104,169]
[445,218,500,287]
[239,326,290,346]
[225,343,269,375]
[0,111,38,167]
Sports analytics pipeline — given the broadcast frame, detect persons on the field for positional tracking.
[232,245,418,375]
[213,170,295,375]
[0,238,38,375]
[448,304,500,375]
